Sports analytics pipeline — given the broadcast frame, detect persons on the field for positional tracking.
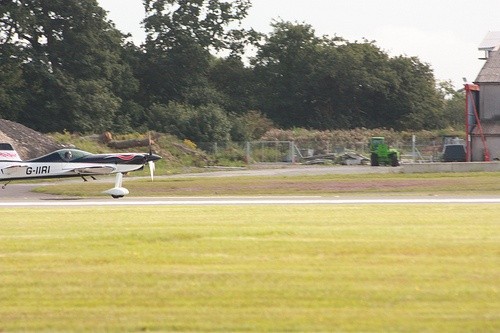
[64,151,73,161]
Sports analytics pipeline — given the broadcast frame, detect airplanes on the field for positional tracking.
[0,139,163,199]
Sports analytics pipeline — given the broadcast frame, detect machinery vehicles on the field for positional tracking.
[369,136,401,167]
